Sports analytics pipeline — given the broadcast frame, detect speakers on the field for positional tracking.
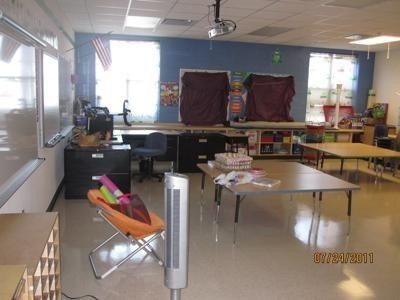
[162,172,190,290]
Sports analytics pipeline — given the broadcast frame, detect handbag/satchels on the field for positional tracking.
[98,193,152,224]
[80,132,100,147]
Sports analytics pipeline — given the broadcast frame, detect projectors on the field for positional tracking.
[208,25,233,39]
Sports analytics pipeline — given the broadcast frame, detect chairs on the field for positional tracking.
[87,188,166,280]
[130,132,168,183]
[91,99,132,126]
[368,126,395,173]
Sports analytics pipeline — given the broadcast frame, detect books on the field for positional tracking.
[253,177,281,187]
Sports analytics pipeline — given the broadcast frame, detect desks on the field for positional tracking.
[299,143,400,175]
[197,142,360,223]
[112,130,181,173]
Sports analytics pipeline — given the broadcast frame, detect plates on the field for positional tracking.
[247,167,270,178]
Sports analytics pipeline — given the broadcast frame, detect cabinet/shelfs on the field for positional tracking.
[0,211,63,300]
[64,143,132,200]
[257,129,365,158]
